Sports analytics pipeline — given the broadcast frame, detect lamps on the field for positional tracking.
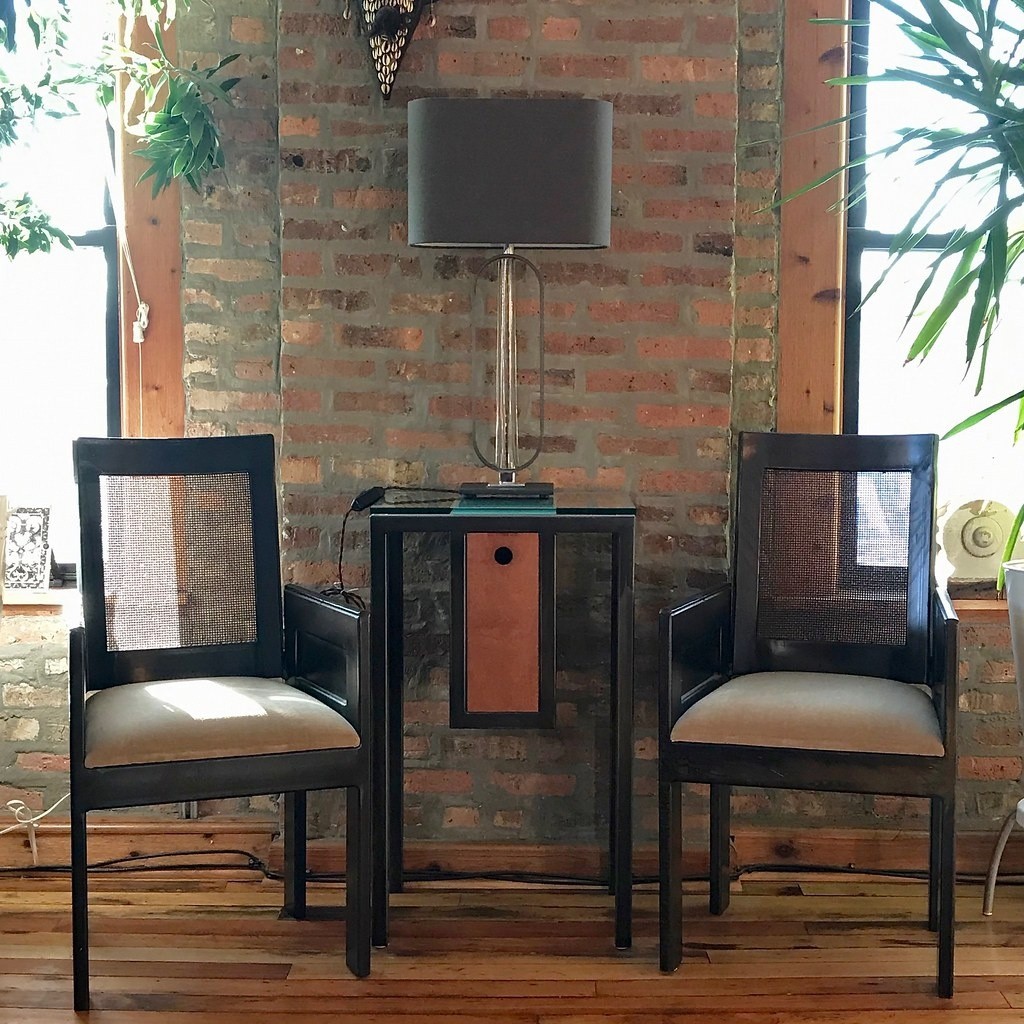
[406,96,612,499]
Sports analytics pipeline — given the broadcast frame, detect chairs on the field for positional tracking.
[73,435,373,1011]
[657,428,959,999]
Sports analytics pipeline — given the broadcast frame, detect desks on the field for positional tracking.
[365,504,638,952]
